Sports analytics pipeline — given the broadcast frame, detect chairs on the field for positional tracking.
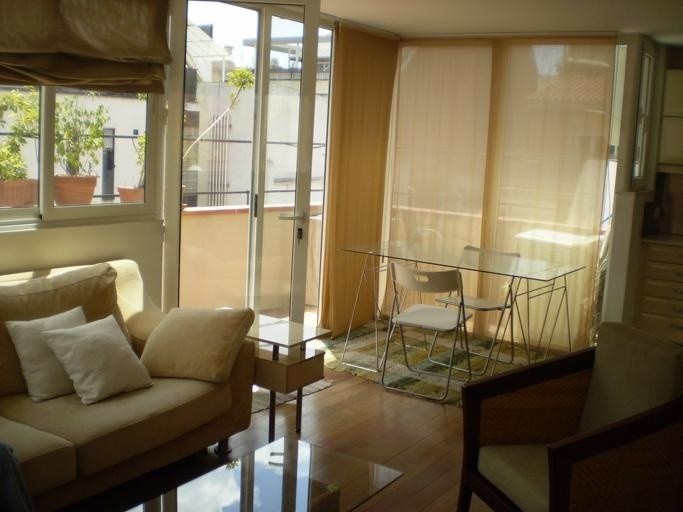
[426,243,521,376]
[457,318,683,512]
[380,260,473,402]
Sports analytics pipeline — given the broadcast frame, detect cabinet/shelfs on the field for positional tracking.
[216,307,332,443]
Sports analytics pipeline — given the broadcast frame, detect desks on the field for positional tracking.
[335,238,587,376]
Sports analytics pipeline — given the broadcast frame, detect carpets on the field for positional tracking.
[319,316,569,410]
[250,376,338,415]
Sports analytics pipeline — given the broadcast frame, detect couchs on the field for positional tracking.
[0,260,256,511]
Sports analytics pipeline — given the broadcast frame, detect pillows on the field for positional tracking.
[3,304,87,403]
[138,305,255,384]
[40,312,157,406]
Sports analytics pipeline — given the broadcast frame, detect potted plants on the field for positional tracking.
[116,93,144,204]
[0,85,41,209]
[53,88,111,207]
